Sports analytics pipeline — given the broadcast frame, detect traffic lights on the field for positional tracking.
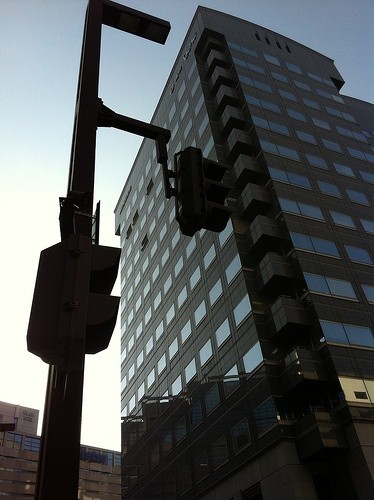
[26,240,121,365]
[174,146,230,236]
